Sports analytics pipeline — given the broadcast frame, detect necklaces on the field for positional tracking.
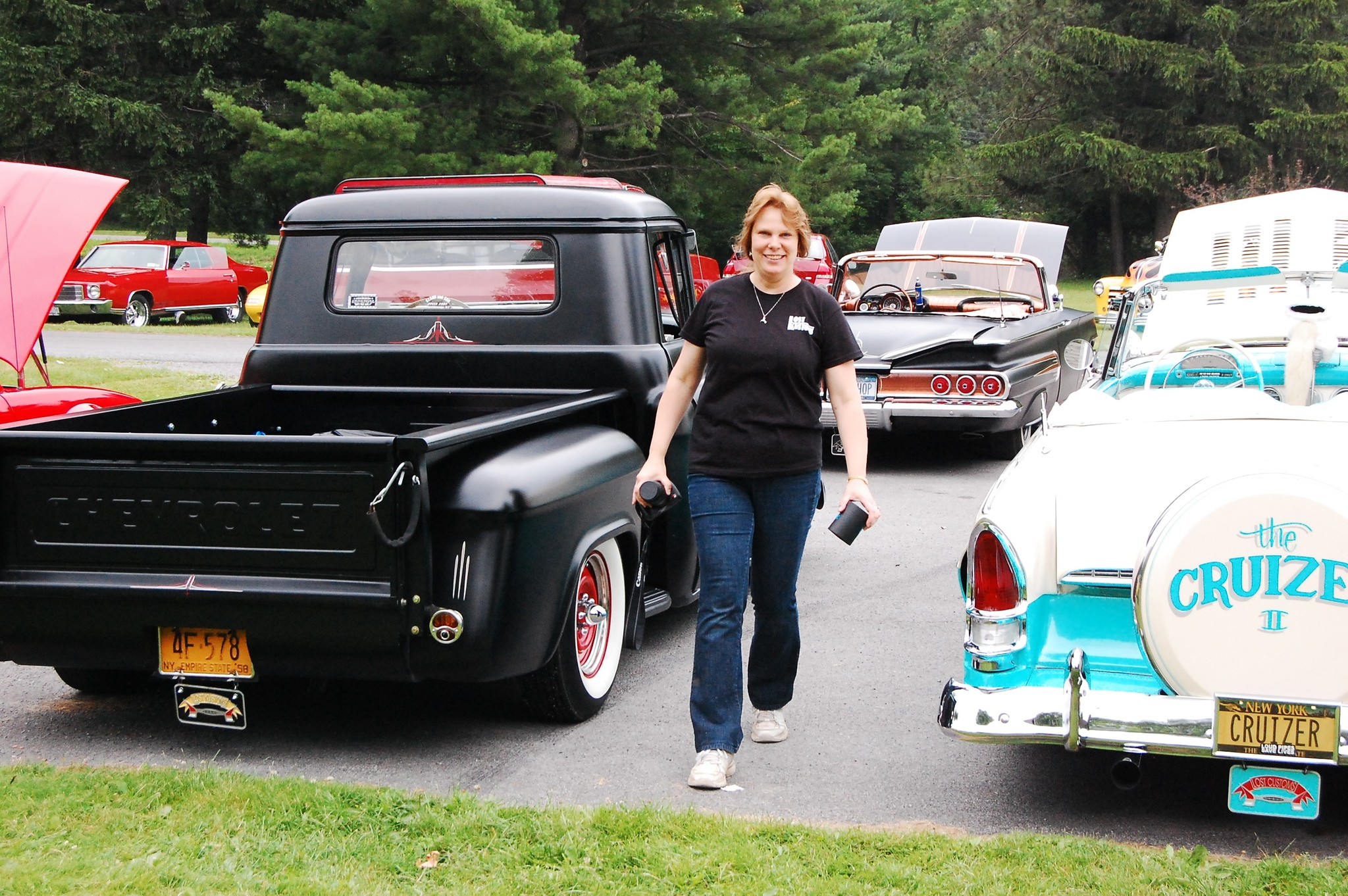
[753,277,793,324]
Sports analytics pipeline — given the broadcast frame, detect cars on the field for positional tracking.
[723,230,842,308]
[1091,237,1172,326]
[820,215,1103,457]
[245,283,269,328]
[0,162,145,431]
[47,238,271,328]
[326,169,724,325]
[934,185,1348,822]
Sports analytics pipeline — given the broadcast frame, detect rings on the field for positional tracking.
[877,507,880,509]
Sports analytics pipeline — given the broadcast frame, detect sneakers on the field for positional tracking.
[687,749,737,788]
[751,709,787,742]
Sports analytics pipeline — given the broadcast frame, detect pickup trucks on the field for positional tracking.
[0,186,714,736]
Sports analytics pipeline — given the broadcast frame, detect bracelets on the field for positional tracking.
[847,476,869,485]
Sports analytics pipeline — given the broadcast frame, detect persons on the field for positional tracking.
[632,182,881,789]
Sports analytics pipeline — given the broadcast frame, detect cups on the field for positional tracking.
[828,500,869,546]
